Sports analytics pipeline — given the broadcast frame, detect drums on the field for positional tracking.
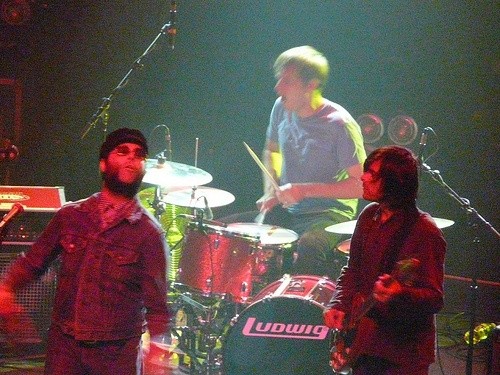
[227,222,300,283]
[334,239,351,274]
[217,276,351,375]
[175,221,261,305]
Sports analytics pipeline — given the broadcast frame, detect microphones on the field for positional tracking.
[168,0,176,49]
[180,214,228,228]
[165,128,172,161]
[0,202,23,231]
[418,128,429,165]
[204,197,213,219]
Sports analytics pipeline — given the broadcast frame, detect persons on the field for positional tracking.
[0,136,19,185]
[0,129,171,375]
[254,46,369,275]
[322,146,447,375]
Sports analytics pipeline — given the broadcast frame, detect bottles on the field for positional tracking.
[464,323,496,344]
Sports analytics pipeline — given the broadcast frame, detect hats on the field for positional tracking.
[98,128,147,158]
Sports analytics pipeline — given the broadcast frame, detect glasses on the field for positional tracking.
[115,144,148,160]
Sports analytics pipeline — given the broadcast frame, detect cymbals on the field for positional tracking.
[325,216,455,235]
[141,159,213,185]
[157,187,235,208]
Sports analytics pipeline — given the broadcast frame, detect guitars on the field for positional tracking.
[328,259,420,375]
